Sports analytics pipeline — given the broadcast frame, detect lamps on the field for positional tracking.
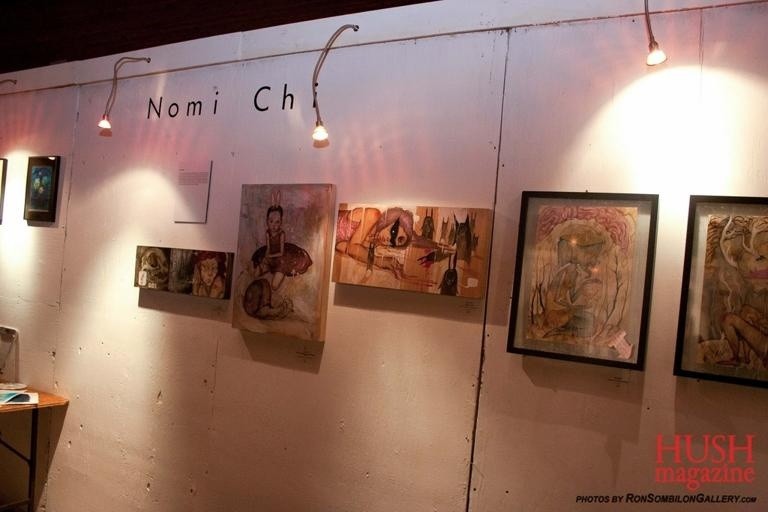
[642,2,667,66]
[310,20,358,144]
[97,55,154,131]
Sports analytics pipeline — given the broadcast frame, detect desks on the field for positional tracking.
[0,386,69,512]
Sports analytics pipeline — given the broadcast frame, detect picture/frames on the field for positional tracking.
[23,154,61,222]
[505,191,660,372]
[672,193,768,391]
[0,158,8,225]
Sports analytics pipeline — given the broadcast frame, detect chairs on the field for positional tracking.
[0,324,20,384]
